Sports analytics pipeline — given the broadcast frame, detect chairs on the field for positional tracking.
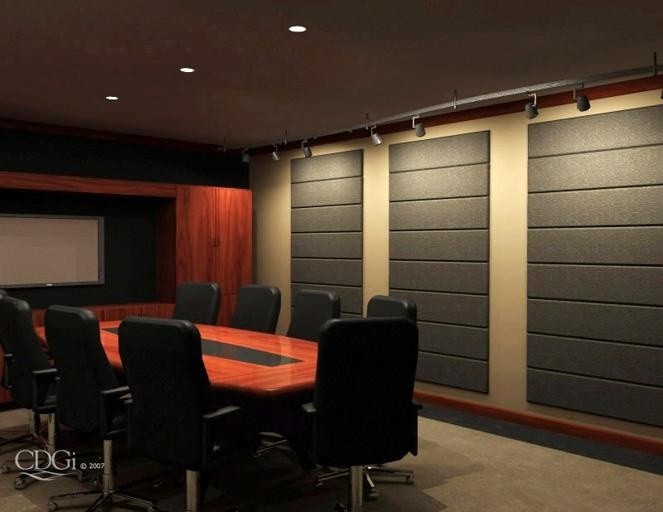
[0,280,422,511]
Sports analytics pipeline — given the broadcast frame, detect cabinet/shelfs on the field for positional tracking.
[156,184,253,301]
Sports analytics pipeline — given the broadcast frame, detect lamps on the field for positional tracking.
[239,81,591,169]
